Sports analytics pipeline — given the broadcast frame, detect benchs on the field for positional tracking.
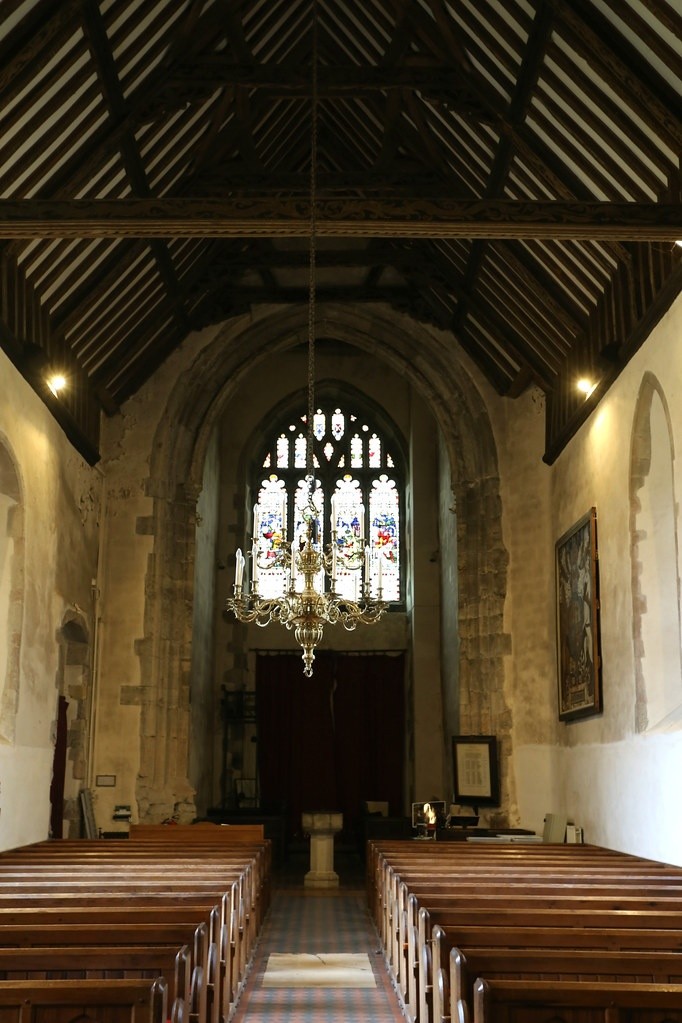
[0,824,272,1023]
[367,835,682,1023]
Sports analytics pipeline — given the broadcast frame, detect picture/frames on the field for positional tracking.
[451,735,500,808]
[554,507,602,721]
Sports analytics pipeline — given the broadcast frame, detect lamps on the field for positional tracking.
[223,0,390,678]
